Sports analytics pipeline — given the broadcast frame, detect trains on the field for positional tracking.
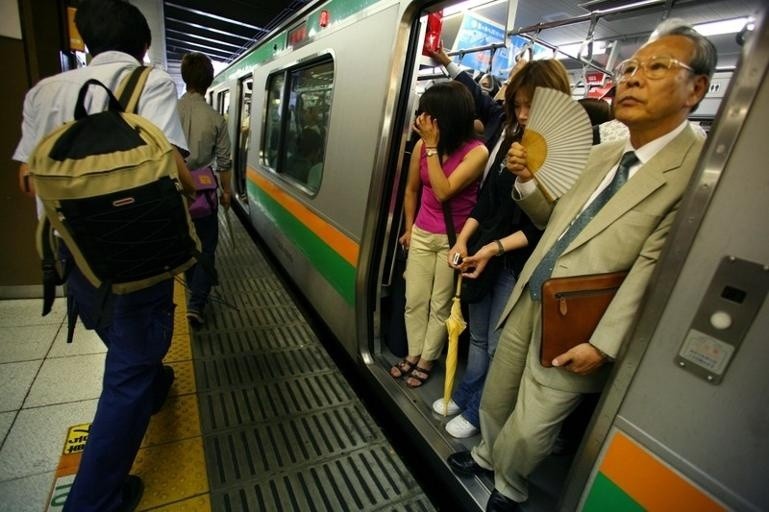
[200,1,768,510]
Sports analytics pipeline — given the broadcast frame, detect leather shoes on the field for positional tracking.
[149,363,174,415]
[446,447,495,477]
[120,474,142,511]
[485,487,523,512]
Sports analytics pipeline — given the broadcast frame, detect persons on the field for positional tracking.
[10,1,196,511]
[390,24,716,512]
[238,74,330,204]
[174,50,236,328]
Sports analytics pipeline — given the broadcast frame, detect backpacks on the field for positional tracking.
[26,66,202,295]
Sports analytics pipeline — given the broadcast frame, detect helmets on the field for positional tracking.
[611,55,696,79]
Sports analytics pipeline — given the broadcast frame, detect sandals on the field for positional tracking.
[391,356,435,388]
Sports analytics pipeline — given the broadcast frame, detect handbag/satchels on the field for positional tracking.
[541,271,630,366]
[184,166,220,217]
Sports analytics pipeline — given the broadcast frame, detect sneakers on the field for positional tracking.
[433,396,462,415]
[445,416,478,440]
[186,306,206,324]
